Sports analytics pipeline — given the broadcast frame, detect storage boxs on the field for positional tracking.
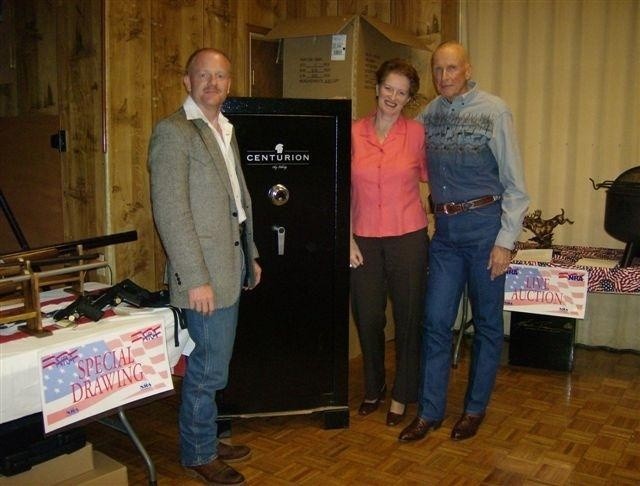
[262,13,433,362]
[0,440,129,486]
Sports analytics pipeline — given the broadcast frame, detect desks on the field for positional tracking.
[0,281,196,486]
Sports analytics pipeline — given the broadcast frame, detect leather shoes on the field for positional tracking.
[450,410,485,440]
[434,195,501,217]
[184,442,252,486]
[359,381,440,441]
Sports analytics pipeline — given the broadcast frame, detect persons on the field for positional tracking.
[147,47,262,486]
[398,41,529,442]
[348,59,431,427]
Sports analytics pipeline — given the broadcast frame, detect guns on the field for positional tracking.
[53,279,151,323]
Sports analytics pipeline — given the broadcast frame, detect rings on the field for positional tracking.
[504,268,508,272]
[349,263,353,268]
